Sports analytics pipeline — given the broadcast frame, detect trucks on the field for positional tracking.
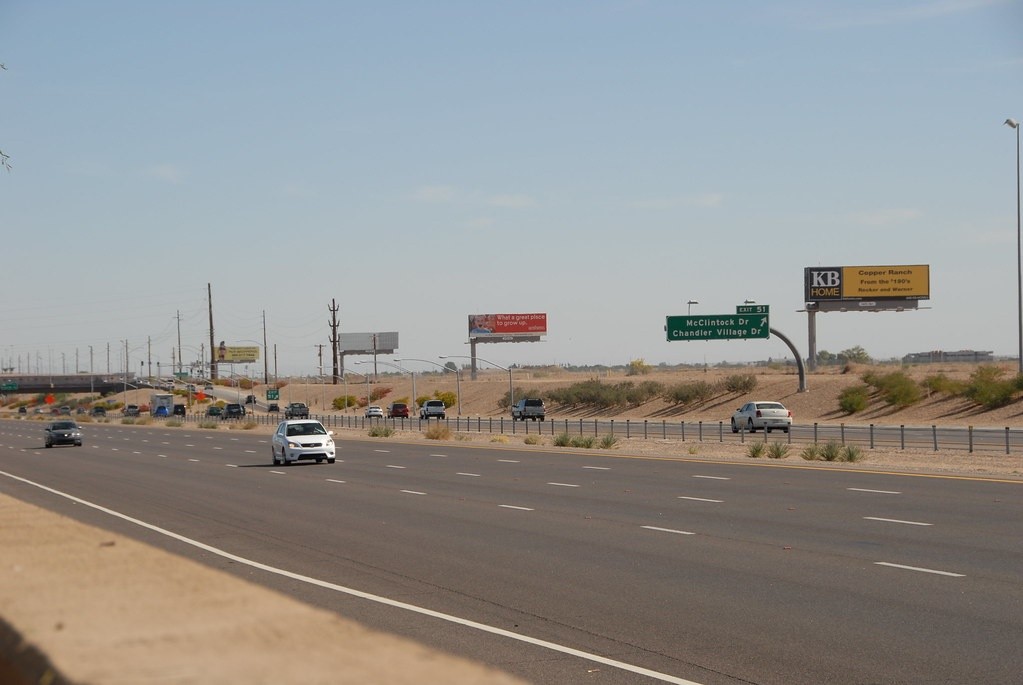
[149,394,175,417]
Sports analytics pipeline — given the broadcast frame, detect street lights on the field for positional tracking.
[315,374,348,414]
[301,376,310,407]
[1003,118,1023,374]
[354,360,417,416]
[394,358,462,415]
[286,376,291,405]
[236,339,269,385]
[178,344,254,414]
[439,356,514,415]
[317,365,371,409]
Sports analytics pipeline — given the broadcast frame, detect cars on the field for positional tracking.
[731,401,793,434]
[43,421,83,448]
[246,395,256,404]
[385,403,409,418]
[18,404,140,418]
[270,419,337,466]
[205,407,224,418]
[364,405,383,419]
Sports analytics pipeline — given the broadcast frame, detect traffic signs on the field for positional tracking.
[665,314,770,340]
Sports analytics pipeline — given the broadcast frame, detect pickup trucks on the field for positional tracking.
[284,402,310,419]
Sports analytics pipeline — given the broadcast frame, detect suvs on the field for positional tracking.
[420,400,446,419]
[174,403,186,417]
[268,403,280,412]
[222,403,242,420]
[511,398,549,421]
